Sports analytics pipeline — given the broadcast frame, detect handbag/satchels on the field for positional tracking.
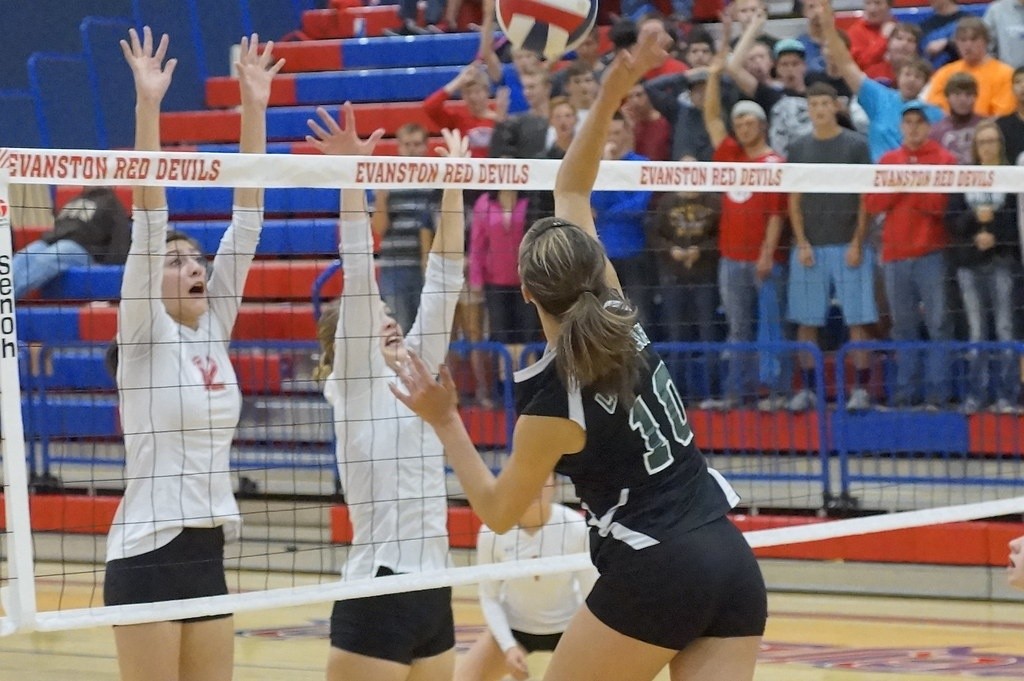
[756,265,792,394]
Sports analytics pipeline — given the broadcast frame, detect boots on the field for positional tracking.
[472,366,498,410]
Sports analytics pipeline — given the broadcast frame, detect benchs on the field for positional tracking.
[0,0,1024,600]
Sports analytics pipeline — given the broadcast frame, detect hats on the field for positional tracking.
[729,100,766,121]
[900,101,928,119]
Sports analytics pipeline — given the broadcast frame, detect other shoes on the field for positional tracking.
[758,394,786,412]
[698,396,742,411]
[787,389,817,413]
[926,400,947,412]
[988,399,1024,415]
[957,395,984,414]
[875,391,924,412]
[846,388,869,410]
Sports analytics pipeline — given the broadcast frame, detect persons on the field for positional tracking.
[306,102,474,681]
[389,30,766,681]
[367,1,1024,414]
[13,185,128,302]
[101,26,289,679]
[1007,535,1024,590]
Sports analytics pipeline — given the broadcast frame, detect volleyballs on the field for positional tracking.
[494,0,600,60]
